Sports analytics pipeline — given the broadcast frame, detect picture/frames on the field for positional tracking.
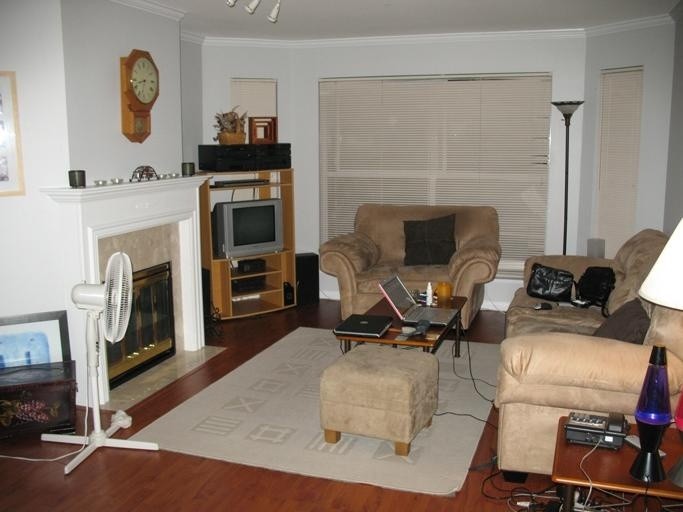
[0,70,26,196]
[0,310,71,368]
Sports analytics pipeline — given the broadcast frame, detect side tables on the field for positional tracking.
[551,415,683,512]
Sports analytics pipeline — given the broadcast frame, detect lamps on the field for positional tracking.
[266,0,281,23]
[224,0,237,7]
[550,100,585,256]
[244,0,262,15]
[636,218,682,486]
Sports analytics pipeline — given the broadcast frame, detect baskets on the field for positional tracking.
[218,112,246,145]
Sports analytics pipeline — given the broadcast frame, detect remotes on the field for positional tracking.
[420,293,438,300]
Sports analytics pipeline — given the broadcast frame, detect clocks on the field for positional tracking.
[119,49,159,144]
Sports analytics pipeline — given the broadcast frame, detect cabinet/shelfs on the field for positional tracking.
[203,170,298,321]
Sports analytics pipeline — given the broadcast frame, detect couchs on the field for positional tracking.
[495,228,683,483]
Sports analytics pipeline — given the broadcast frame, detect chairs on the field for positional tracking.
[318,203,502,331]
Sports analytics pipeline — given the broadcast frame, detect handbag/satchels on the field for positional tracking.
[527,262,574,302]
[576,267,615,302]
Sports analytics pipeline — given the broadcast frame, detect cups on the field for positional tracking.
[436,283,451,305]
[181,162,194,176]
[67,170,85,188]
[95,173,178,186]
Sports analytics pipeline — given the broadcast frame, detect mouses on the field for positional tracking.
[534,302,552,310]
[416,320,430,333]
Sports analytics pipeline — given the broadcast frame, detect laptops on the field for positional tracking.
[379,272,459,327]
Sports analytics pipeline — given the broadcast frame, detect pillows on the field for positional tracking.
[591,298,651,345]
[401,214,456,267]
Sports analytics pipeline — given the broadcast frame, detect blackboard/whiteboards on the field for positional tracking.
[563,412,628,450]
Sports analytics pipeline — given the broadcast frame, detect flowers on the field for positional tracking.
[212,106,248,133]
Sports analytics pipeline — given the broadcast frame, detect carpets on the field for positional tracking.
[126,327,502,495]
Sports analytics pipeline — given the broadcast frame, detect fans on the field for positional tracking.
[38,252,159,475]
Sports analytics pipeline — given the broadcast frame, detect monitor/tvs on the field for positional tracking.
[211,198,284,257]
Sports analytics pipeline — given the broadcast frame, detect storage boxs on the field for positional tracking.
[0,360,77,444]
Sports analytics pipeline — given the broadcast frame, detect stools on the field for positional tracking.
[319,344,440,456]
